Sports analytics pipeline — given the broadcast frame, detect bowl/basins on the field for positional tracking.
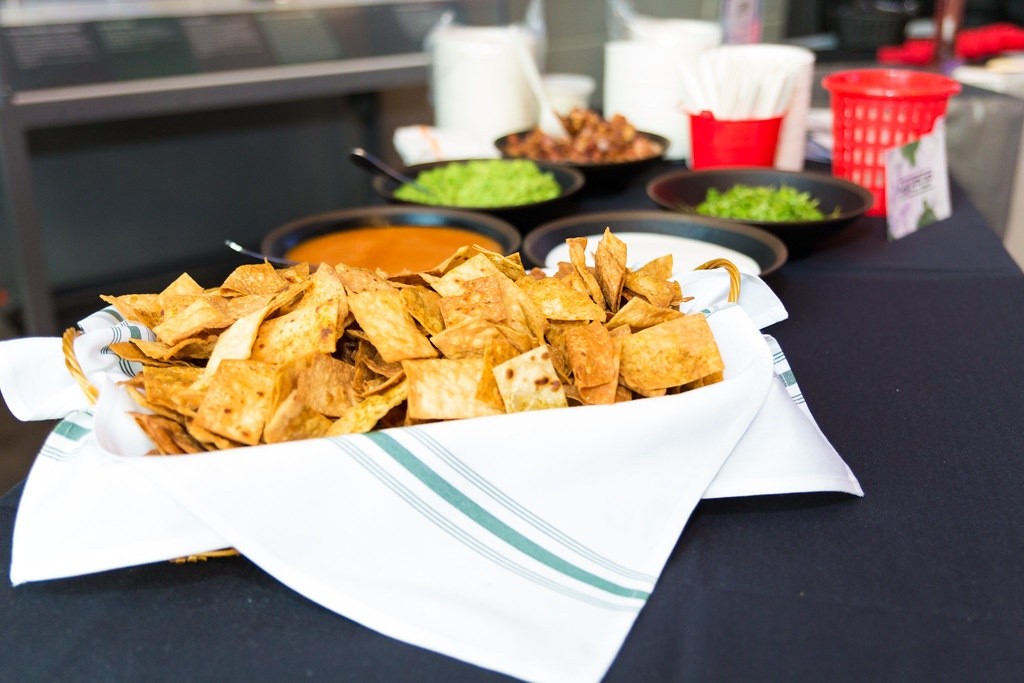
[540,72,596,113]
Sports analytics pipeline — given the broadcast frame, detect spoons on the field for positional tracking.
[505,25,574,146]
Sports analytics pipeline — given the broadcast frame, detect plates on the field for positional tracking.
[646,170,875,235]
[260,208,522,264]
[493,130,672,189]
[371,160,586,226]
[518,212,787,277]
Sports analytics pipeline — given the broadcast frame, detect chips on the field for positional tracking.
[100,226,724,454]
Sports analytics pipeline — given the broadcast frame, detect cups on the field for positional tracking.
[430,22,537,137]
[604,18,815,170]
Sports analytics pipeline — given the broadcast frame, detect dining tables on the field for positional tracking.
[2,137,1024,682]
